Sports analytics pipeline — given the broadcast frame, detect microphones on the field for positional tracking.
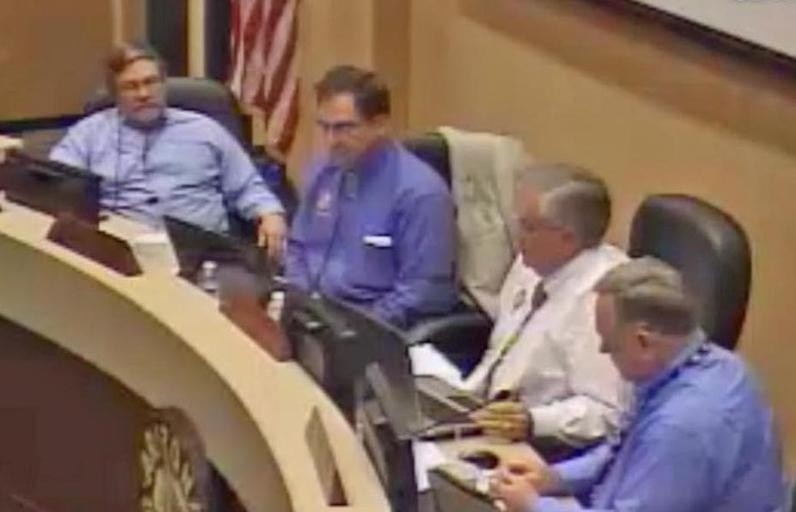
[422,388,515,426]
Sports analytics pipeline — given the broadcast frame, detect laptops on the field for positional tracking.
[162,213,276,311]
[323,291,485,444]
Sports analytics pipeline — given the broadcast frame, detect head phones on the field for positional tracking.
[105,54,167,98]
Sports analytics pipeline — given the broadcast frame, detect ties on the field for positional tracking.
[480,280,547,399]
[584,338,712,502]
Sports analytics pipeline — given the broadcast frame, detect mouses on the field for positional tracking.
[460,445,505,470]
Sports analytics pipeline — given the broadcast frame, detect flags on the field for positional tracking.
[225,0,300,154]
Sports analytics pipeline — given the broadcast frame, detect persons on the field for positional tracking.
[460,160,638,451]
[45,35,289,264]
[490,255,786,512]
[279,64,457,334]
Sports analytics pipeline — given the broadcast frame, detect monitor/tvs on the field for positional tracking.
[355,448,495,511]
[1,143,110,228]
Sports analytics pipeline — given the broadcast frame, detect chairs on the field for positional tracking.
[626,193,752,350]
[82,77,283,268]
[395,133,512,355]
